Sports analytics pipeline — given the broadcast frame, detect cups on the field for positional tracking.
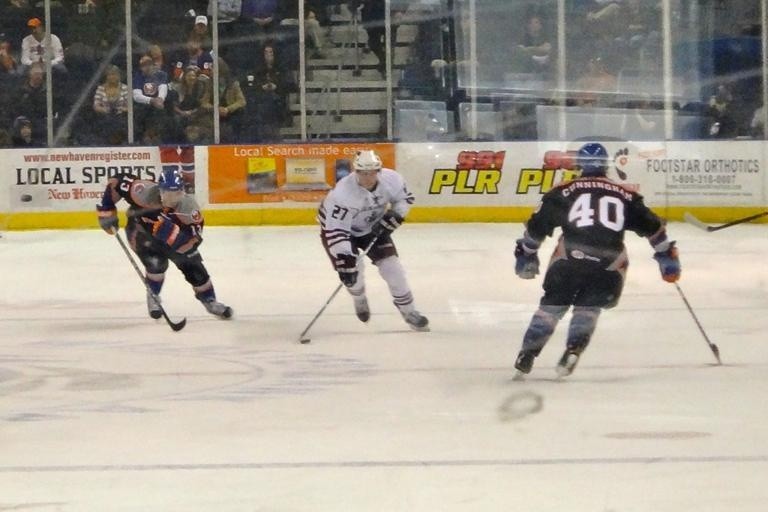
[247,76,254,87]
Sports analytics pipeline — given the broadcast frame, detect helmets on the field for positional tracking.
[159,170,183,191]
[577,144,608,170]
[353,150,382,171]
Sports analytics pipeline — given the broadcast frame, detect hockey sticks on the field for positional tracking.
[673,278,719,364]
[300,228,384,343]
[683,209,767,233]
[109,224,186,332]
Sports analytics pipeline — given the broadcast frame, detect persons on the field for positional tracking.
[96,169,234,319]
[317,150,429,328]
[1,0,411,147]
[514,143,681,374]
[406,0,767,140]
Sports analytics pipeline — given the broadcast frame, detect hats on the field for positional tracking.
[28,18,41,27]
[195,15,208,26]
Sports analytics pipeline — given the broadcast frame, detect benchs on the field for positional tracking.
[278,24,419,139]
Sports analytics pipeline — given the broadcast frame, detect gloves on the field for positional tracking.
[372,211,403,239]
[654,241,681,282]
[152,216,182,248]
[515,237,539,278]
[335,252,358,286]
[96,204,119,234]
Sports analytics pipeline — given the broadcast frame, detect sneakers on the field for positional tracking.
[515,349,535,374]
[353,294,369,322]
[203,300,233,318]
[401,311,428,328]
[559,347,579,377]
[146,291,164,319]
